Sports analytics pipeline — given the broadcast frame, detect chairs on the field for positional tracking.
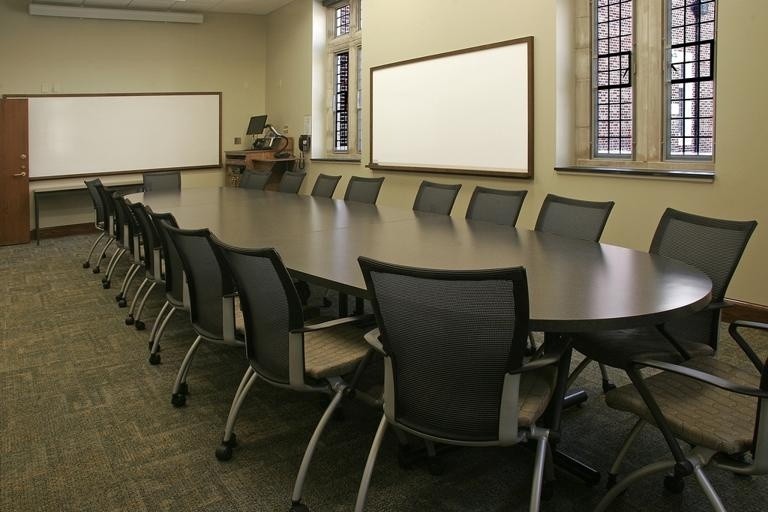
[343,176,385,204]
[535,194,616,242]
[238,169,272,189]
[595,317,768,511]
[466,186,528,226]
[565,207,759,489]
[85,178,332,446]
[311,173,343,199]
[412,180,462,215]
[355,256,566,512]
[208,232,429,512]
[272,171,307,193]
[143,171,181,190]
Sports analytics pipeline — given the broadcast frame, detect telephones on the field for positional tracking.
[299,134,310,152]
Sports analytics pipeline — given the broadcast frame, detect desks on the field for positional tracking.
[123,187,711,484]
[33,179,146,245]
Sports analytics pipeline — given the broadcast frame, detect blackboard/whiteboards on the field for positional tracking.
[3,92,223,181]
[365,35,535,180]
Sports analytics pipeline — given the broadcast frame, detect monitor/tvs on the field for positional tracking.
[246,115,267,135]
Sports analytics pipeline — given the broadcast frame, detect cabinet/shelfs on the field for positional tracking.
[222,149,304,190]
